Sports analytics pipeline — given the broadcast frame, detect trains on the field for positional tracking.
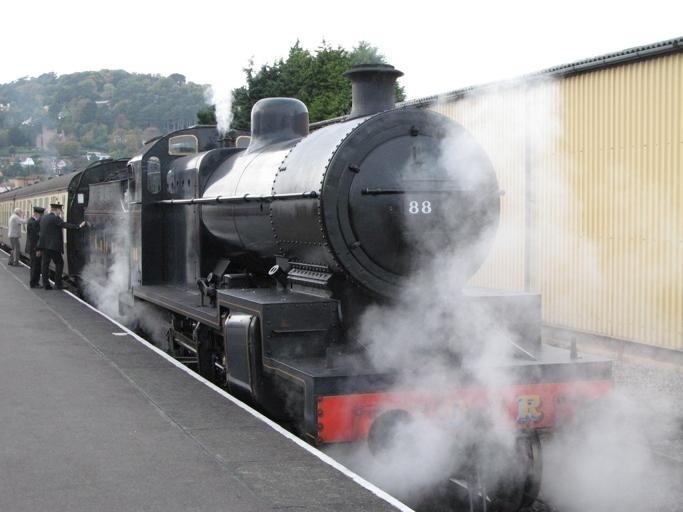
[0,64,616,512]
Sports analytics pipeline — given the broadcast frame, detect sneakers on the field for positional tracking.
[30,284,69,291]
[8,262,23,267]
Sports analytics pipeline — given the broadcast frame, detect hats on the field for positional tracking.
[50,203,63,208]
[32,206,45,214]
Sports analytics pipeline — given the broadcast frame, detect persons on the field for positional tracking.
[25,204,84,290]
[8,208,27,267]
